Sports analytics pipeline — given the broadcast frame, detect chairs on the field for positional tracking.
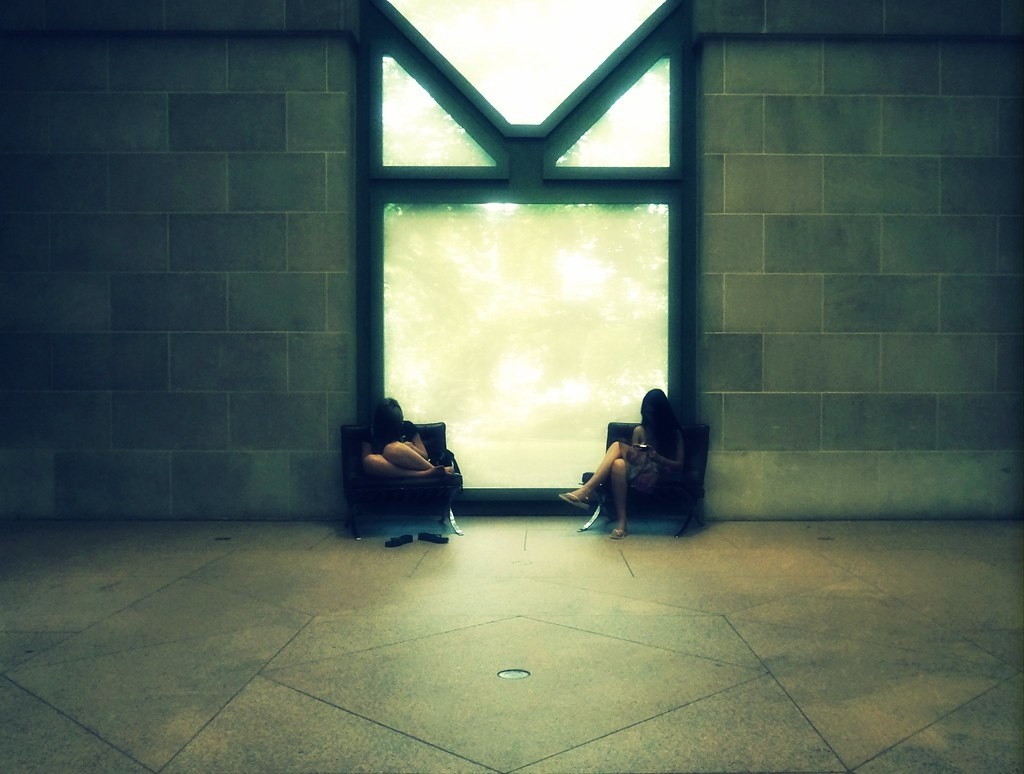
[341,422,465,541]
[577,423,710,539]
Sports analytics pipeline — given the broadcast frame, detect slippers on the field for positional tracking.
[608,529,628,539]
[385,535,413,547]
[559,492,590,511]
[418,533,448,543]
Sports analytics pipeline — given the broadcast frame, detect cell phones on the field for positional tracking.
[640,445,648,449]
[401,435,406,442]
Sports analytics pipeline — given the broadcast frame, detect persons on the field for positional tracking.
[560,389,684,539]
[362,398,455,477]
